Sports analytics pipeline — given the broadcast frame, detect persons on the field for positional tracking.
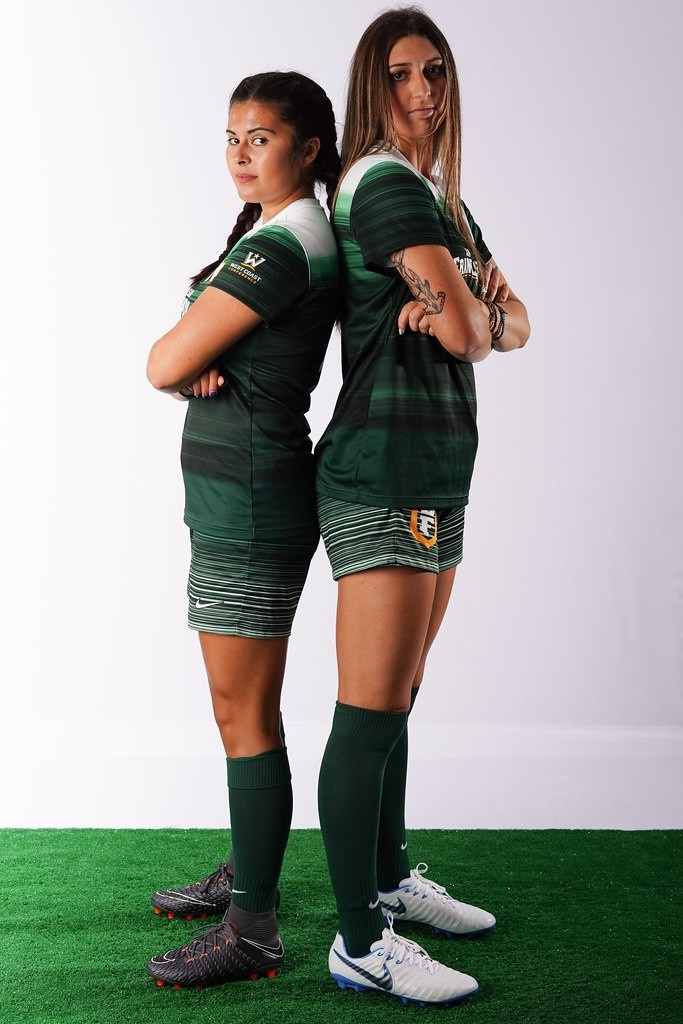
[316,3,531,1004]
[147,71,343,993]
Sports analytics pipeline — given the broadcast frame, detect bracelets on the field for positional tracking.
[178,391,195,399]
[474,295,507,341]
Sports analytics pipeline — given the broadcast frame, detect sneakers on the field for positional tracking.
[146,923,286,993]
[150,864,279,915]
[377,867,497,941]
[328,928,478,1005]
